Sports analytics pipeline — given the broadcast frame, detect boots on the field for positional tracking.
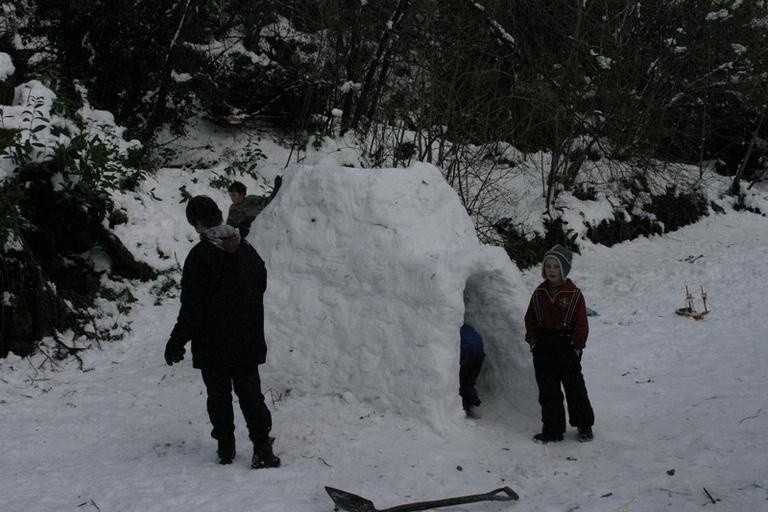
[216,430,236,465]
[248,432,281,469]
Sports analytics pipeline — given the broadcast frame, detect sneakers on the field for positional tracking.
[533,430,565,444]
[577,427,594,442]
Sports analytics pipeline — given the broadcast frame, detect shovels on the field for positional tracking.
[324,486,519,512]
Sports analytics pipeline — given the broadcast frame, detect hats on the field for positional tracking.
[542,244,573,281]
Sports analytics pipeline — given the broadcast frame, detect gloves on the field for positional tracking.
[164,337,186,366]
[274,174,283,189]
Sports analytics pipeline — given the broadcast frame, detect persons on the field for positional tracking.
[525,244,595,443]
[163,194,280,470]
[459,323,487,419]
[225,174,283,239]
